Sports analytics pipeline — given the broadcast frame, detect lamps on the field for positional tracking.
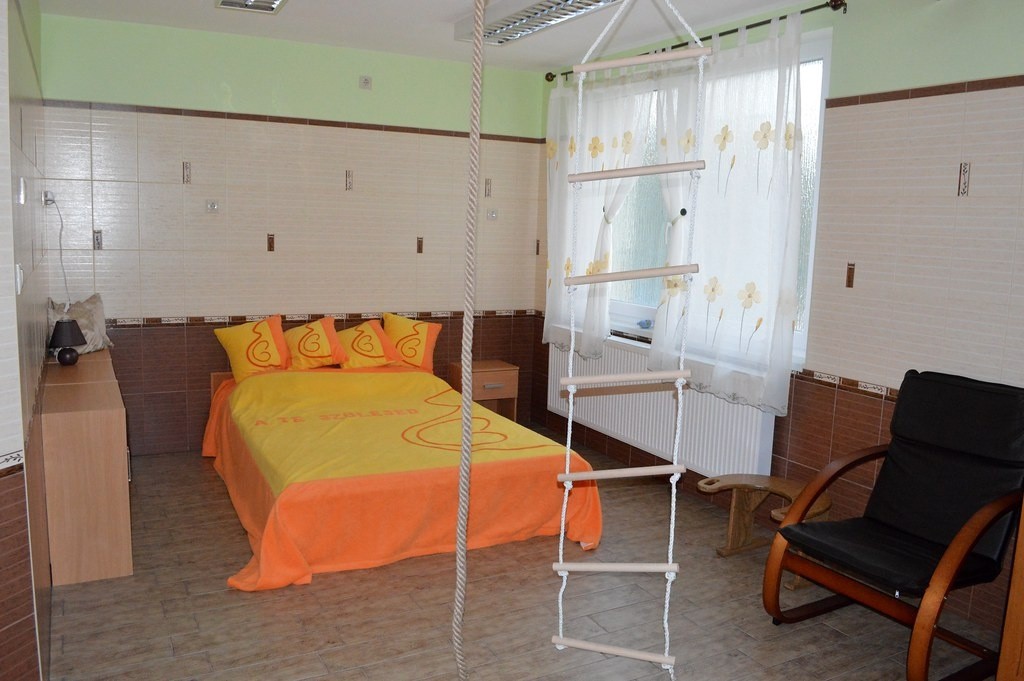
[48,318,89,365]
[453,0,622,48]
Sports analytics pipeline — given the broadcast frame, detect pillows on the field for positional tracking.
[336,320,404,370]
[53,293,116,348]
[213,314,293,385]
[382,312,443,375]
[283,316,350,370]
[44,301,108,356]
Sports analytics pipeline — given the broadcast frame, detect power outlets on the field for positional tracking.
[207,199,219,213]
[42,190,48,206]
[488,208,499,221]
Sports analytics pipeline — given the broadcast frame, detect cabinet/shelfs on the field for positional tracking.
[38,349,136,588]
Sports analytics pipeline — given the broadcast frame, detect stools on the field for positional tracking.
[699,474,834,590]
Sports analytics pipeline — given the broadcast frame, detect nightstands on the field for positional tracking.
[447,359,520,422]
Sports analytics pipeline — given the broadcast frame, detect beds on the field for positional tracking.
[199,368,603,593]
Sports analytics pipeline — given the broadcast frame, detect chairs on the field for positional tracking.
[762,369,1024,681]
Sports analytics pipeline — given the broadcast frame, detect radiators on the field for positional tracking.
[547,323,779,480]
